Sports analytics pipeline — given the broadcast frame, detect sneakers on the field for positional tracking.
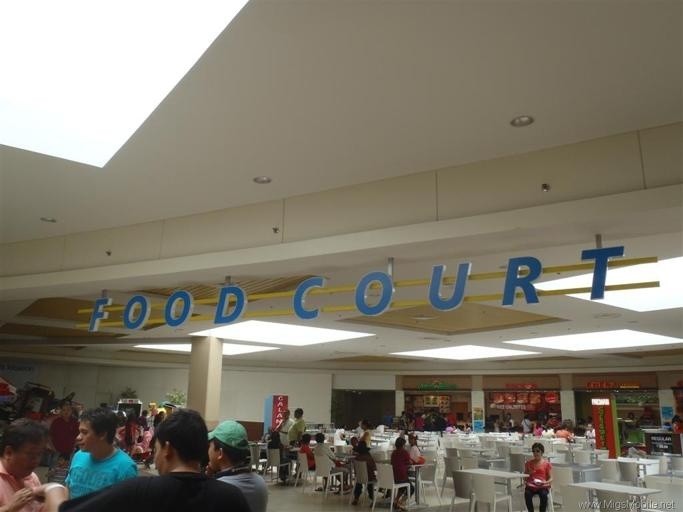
[393,498,407,511]
[352,499,358,505]
[339,483,352,491]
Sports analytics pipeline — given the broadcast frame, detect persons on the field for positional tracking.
[0,382,683,512]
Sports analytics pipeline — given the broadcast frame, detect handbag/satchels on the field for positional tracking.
[526,480,551,491]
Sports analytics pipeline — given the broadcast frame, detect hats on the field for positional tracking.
[208,421,250,451]
[407,433,418,439]
